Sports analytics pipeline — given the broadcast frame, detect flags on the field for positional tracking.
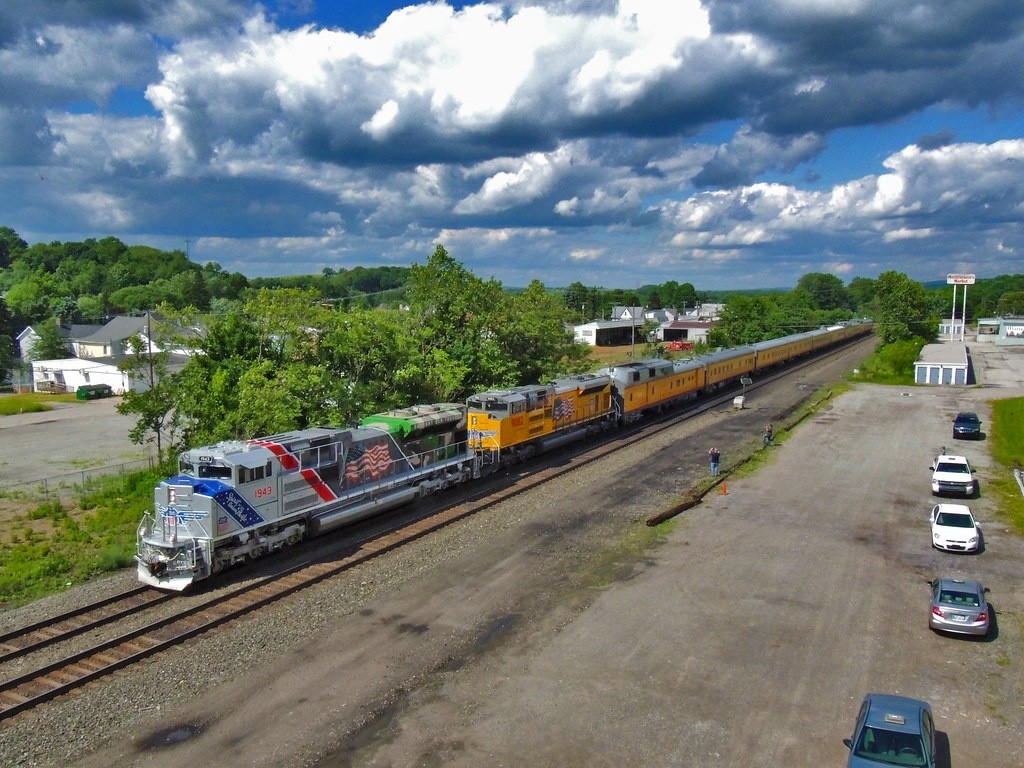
[344,438,393,485]
[552,396,575,422]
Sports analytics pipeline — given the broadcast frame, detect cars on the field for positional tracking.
[929,455,977,498]
[926,578,991,636]
[840,692,938,768]
[929,503,981,553]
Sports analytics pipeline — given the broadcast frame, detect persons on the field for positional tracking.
[709,447,721,477]
[762,424,772,444]
[406,448,421,469]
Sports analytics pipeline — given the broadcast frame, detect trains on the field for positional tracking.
[132,317,874,596]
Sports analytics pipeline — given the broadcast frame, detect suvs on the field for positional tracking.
[952,411,982,440]
